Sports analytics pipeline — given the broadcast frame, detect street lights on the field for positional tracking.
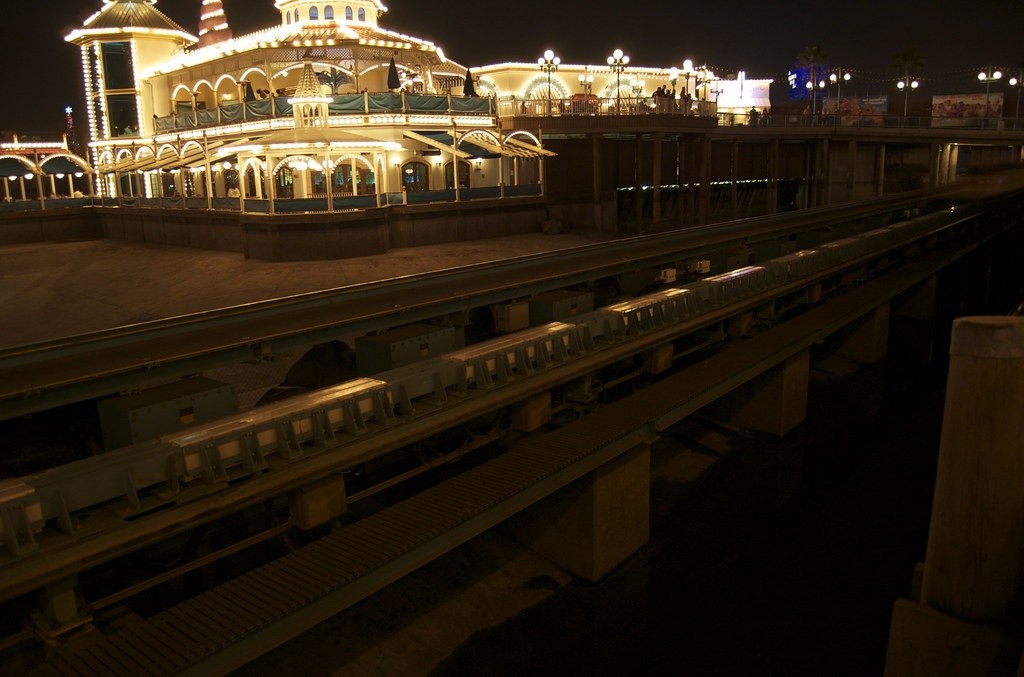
[829,73,851,127]
[538,49,562,115]
[669,58,704,118]
[978,71,1003,128]
[605,48,630,115]
[805,80,826,126]
[897,81,916,123]
[577,66,593,115]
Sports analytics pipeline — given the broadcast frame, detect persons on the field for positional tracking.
[802,104,837,127]
[749,106,774,128]
[652,84,695,116]
[557,100,565,117]
[824,98,884,126]
[173,190,180,199]
[932,100,1001,126]
[520,102,527,114]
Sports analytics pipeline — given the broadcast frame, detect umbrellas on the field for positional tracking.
[462,68,475,97]
[245,78,255,102]
[387,55,401,94]
